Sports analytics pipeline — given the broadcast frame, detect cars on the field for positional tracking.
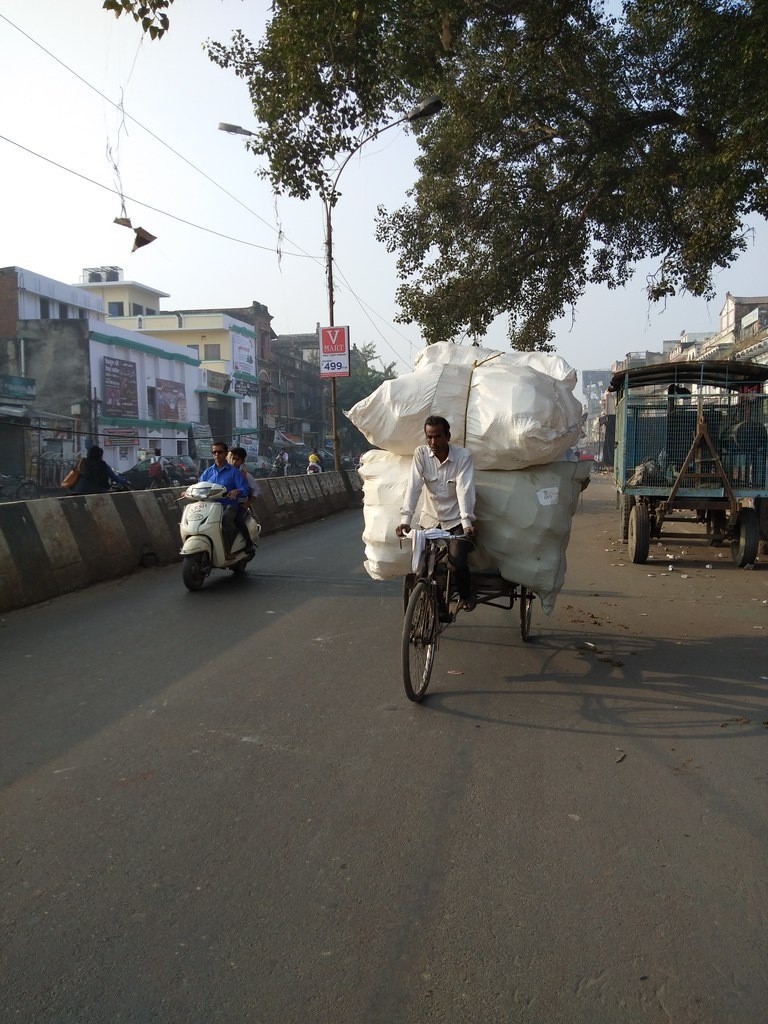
[293,448,335,469]
[576,448,598,462]
[119,454,199,491]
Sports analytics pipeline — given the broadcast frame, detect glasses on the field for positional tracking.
[212,451,226,454]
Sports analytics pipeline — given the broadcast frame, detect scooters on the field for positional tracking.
[176,482,261,592]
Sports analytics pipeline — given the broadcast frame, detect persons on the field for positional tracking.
[395,415,479,612]
[66,446,127,496]
[349,447,358,459]
[306,447,324,473]
[198,442,260,560]
[151,449,173,487]
[265,446,288,476]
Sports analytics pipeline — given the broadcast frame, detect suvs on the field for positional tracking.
[244,455,269,478]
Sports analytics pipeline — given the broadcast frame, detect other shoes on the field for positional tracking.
[224,549,231,560]
[244,541,252,554]
[461,587,477,613]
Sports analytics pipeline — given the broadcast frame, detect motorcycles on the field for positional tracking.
[308,465,318,475]
[268,456,286,478]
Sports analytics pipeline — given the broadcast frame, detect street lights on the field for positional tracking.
[218,96,444,472]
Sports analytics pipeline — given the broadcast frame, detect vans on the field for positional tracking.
[193,456,215,475]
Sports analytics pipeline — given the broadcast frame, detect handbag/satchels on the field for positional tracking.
[60,458,85,488]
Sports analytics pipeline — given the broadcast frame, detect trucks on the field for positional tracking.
[606,360,768,570]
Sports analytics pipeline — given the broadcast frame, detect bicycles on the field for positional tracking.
[0,474,40,501]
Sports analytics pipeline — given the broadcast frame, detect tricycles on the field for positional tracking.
[398,526,536,703]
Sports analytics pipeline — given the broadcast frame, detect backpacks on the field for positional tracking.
[149,456,162,479]
